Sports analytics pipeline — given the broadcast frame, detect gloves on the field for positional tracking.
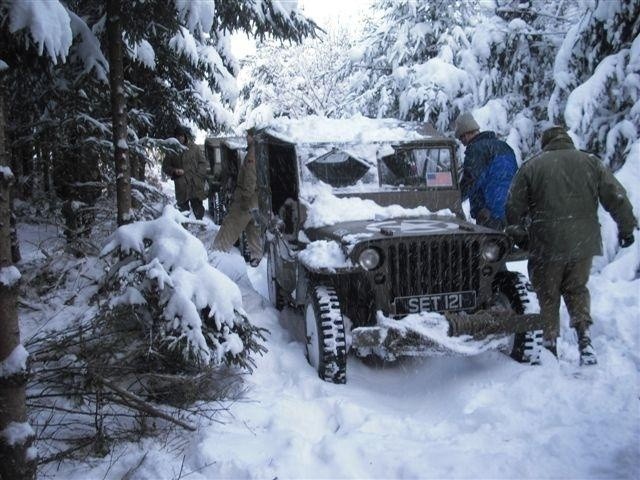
[616,230,634,248]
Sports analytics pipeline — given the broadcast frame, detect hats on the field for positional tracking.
[541,127,569,150]
[454,111,480,140]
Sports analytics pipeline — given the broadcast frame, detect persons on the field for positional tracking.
[209,125,272,268]
[451,109,520,228]
[161,125,211,221]
[500,124,635,366]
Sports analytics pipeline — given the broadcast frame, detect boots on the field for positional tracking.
[575,320,598,366]
